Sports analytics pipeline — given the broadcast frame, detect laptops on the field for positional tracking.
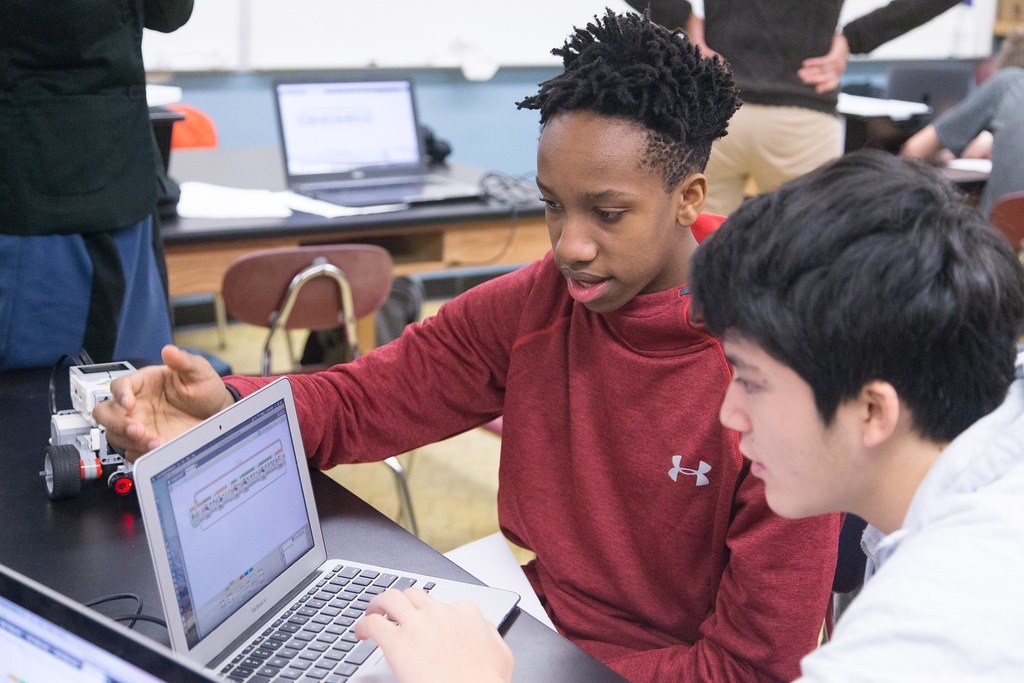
[273,77,485,208]
[0,565,222,683]
[132,376,522,683]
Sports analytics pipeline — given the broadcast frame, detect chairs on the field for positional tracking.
[220,244,422,540]
[989,193,1023,264]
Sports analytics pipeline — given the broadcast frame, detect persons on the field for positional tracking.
[91,1,844,683]
[621,1,965,217]
[0,0,195,370]
[354,149,1024,683]
[896,31,1024,221]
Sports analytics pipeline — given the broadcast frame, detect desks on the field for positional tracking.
[1,359,631,683]
[163,185,553,355]
